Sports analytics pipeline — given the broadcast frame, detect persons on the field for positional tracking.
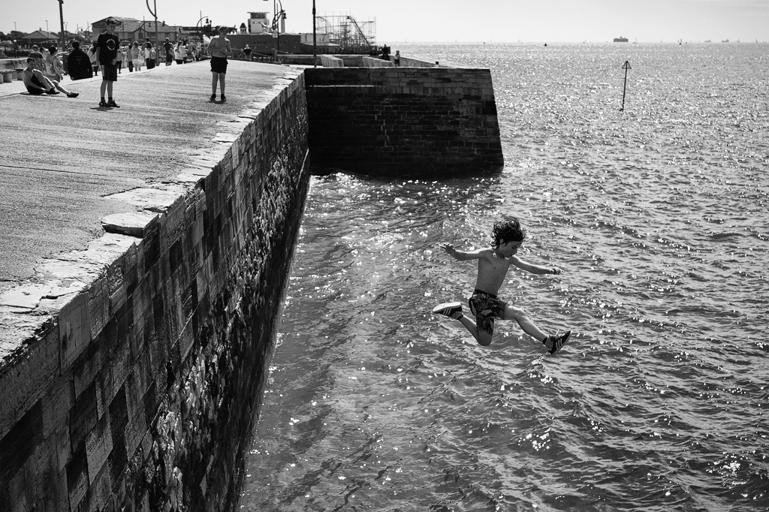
[23,58,79,99]
[27,38,208,80]
[96,19,119,109]
[208,29,231,101]
[432,220,572,355]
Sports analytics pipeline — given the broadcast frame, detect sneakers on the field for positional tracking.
[432,301,462,317]
[210,94,226,101]
[46,89,60,95]
[98,100,120,108]
[67,91,79,98]
[547,330,572,355]
